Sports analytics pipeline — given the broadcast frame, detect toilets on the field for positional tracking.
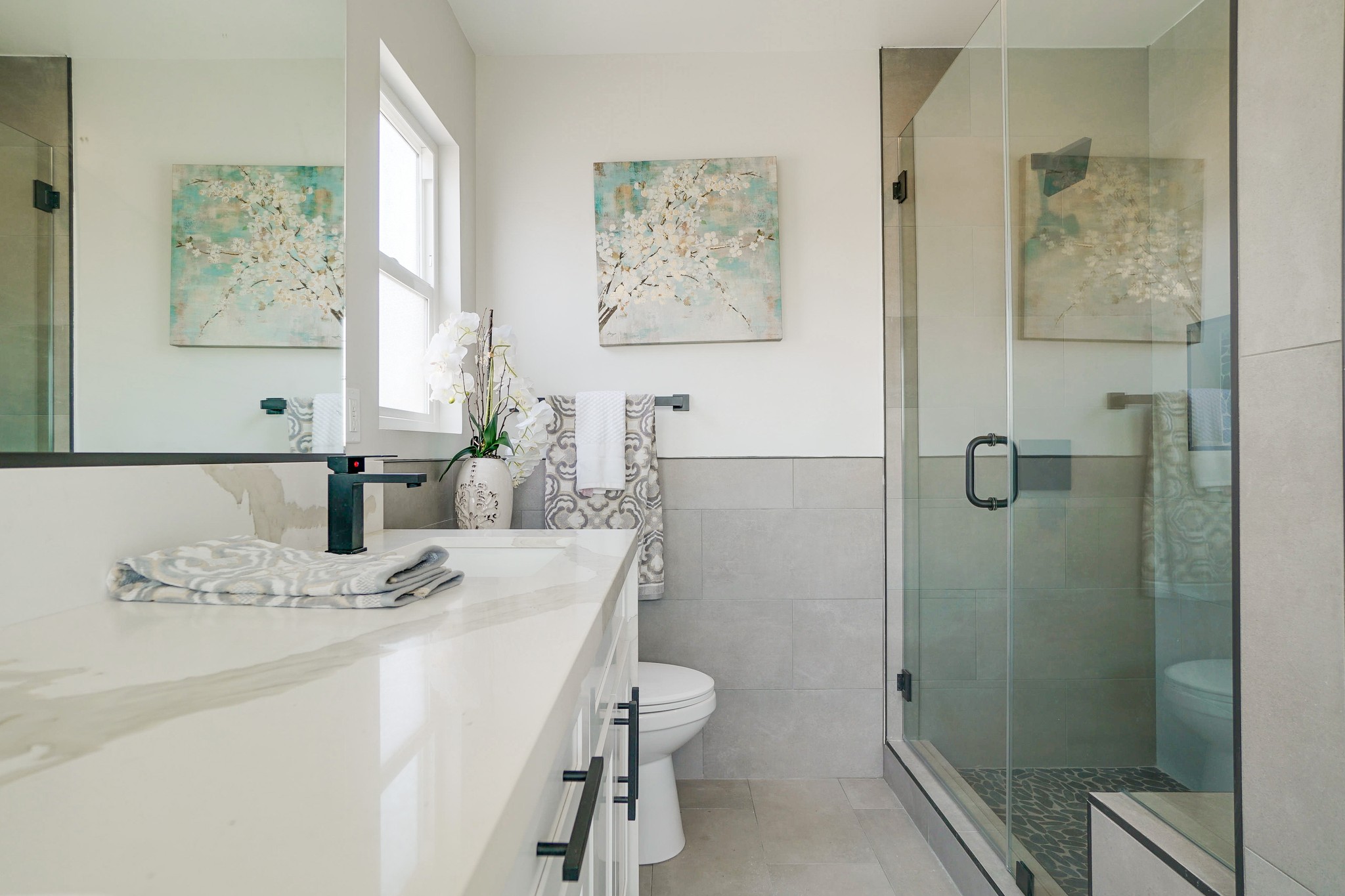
[1162,655,1238,792]
[638,660,719,866]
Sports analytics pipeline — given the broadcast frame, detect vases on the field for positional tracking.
[454,457,512,530]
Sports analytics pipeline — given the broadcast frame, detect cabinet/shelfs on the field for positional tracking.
[530,606,638,896]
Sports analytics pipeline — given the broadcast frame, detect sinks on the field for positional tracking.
[382,532,575,578]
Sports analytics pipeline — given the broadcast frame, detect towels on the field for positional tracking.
[544,393,666,600]
[287,397,313,452]
[573,390,629,492]
[1190,389,1231,494]
[105,536,463,607]
[1138,388,1236,602]
[313,393,345,454]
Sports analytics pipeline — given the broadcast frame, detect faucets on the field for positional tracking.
[321,454,430,554]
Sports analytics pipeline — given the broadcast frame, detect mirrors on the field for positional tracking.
[0,0,352,470]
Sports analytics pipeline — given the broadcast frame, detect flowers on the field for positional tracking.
[427,307,548,488]
